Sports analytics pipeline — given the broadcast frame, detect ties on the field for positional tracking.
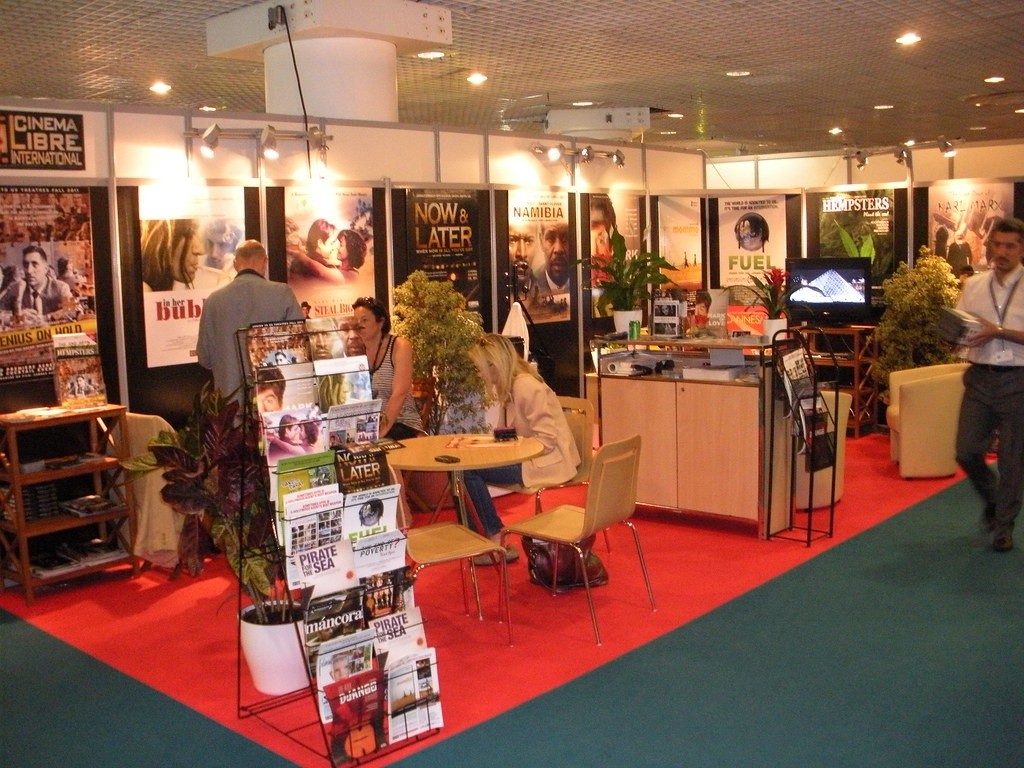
[32,289,39,312]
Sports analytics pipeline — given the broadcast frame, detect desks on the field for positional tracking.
[383,434,544,620]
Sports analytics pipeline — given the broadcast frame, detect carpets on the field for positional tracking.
[0,430,999,768]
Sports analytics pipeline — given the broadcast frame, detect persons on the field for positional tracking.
[331,653,350,681]
[956,222,1024,550]
[590,194,626,272]
[319,375,348,408]
[274,352,286,365]
[308,318,336,359]
[335,317,365,358]
[695,291,712,317]
[268,420,318,454]
[72,376,96,397]
[258,369,286,413]
[139,219,238,294]
[352,296,424,439]
[655,304,676,334]
[464,335,580,565]
[0,193,96,327]
[509,223,570,290]
[196,240,304,549]
[269,415,299,462]
[292,218,366,284]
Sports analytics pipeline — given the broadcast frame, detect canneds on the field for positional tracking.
[629,321,641,340]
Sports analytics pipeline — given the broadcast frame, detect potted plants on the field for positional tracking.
[118,380,310,696]
[390,269,498,509]
[566,227,681,336]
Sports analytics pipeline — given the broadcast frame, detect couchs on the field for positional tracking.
[792,392,854,513]
[885,367,966,477]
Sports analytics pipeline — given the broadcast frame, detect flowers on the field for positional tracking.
[733,267,813,320]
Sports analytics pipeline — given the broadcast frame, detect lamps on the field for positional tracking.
[548,144,626,168]
[854,136,958,171]
[262,126,334,159]
[199,124,221,158]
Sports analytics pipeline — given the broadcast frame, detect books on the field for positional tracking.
[55,332,106,408]
[247,316,444,755]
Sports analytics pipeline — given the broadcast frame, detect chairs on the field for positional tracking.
[116,377,654,648]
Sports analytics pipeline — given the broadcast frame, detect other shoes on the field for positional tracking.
[469,543,519,567]
[992,521,1014,552]
[981,501,998,532]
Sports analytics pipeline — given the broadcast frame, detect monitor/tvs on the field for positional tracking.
[785,256,871,326]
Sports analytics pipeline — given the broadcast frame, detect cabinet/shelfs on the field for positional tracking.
[0,403,141,617]
[232,318,447,768]
[806,324,879,433]
[595,336,797,540]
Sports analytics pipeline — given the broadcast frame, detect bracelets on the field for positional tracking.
[996,326,1004,339]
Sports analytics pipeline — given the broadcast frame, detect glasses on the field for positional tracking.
[356,296,384,313]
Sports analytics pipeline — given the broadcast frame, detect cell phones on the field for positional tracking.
[435,455,460,464]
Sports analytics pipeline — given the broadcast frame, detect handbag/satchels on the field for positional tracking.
[521,531,609,594]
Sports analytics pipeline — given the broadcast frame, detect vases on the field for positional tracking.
[761,318,787,348]
